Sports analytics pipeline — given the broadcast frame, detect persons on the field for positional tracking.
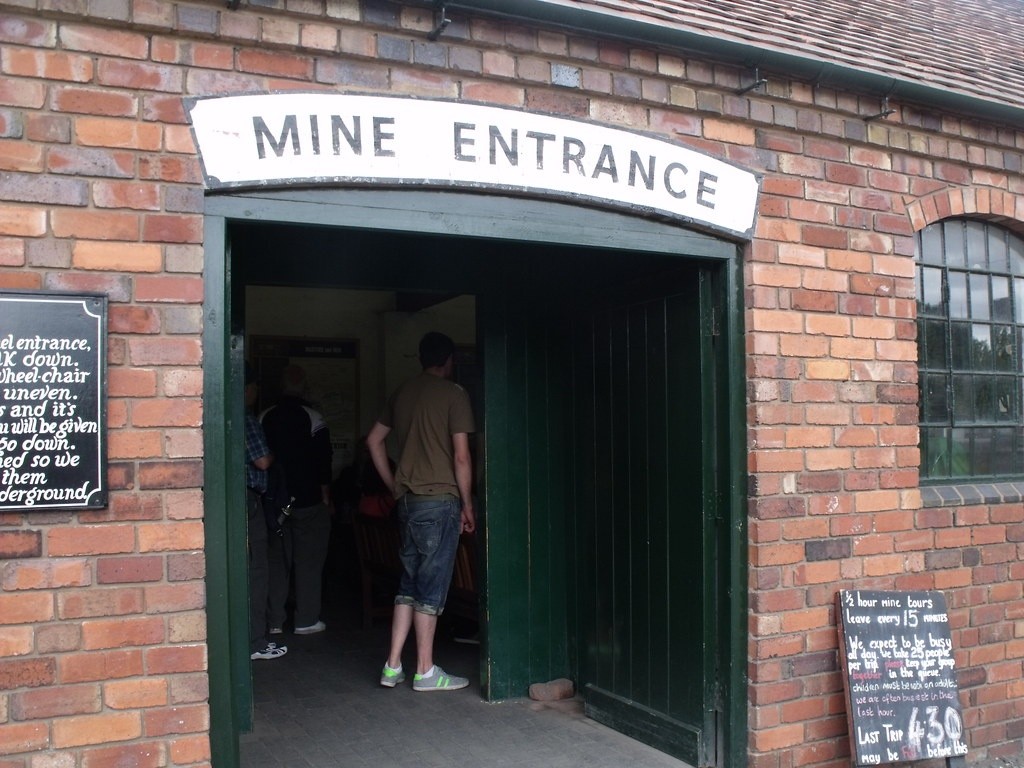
[368,332,477,694]
[244,371,287,660]
[257,366,336,636]
[343,437,397,524]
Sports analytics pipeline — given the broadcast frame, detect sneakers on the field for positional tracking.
[412,665,468,692]
[293,621,326,635]
[268,624,284,635]
[250,644,289,659]
[381,662,409,688]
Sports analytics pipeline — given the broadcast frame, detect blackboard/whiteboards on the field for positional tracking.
[0,288,108,512]
[832,589,969,766]
